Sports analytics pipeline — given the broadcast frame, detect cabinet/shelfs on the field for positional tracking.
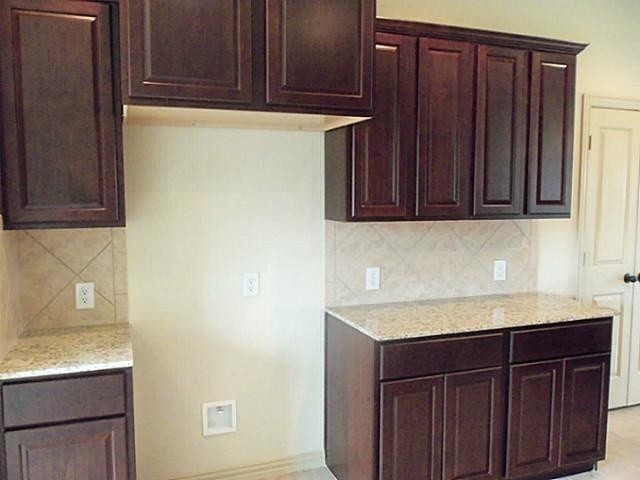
[375,322,615,480]
[352,32,578,221]
[0,2,119,225]
[118,1,376,109]
[1,372,130,480]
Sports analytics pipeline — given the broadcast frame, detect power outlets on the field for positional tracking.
[74,281,96,311]
[243,272,260,297]
